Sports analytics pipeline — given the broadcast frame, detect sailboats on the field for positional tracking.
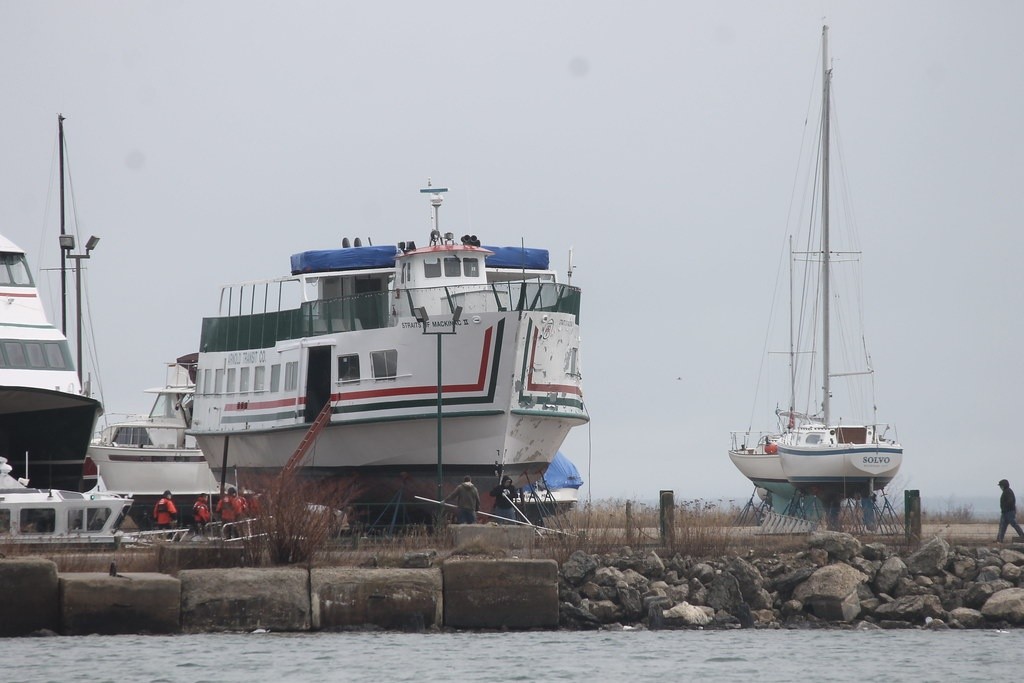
[726,25,904,527]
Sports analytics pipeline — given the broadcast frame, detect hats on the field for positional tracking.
[227,486,236,495]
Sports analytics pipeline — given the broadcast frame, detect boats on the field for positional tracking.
[1,458,188,561]
[1,114,108,494]
[88,346,583,549]
[179,172,592,523]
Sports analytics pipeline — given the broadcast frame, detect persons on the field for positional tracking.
[992,479,1024,544]
[154,489,178,541]
[490,475,518,525]
[440,475,480,524]
[194,487,269,538]
